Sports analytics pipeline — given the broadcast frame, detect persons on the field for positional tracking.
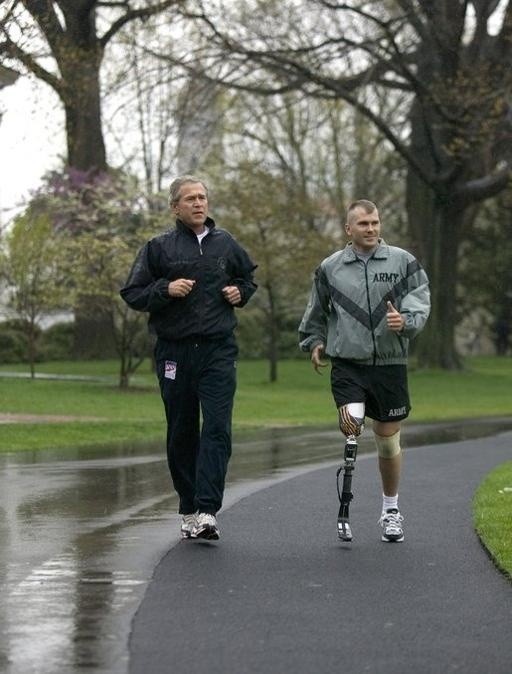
[298,199,433,542]
[118,175,260,542]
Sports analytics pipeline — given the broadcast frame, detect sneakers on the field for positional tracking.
[379,507,404,543]
[190,513,221,540]
[180,512,197,541]
